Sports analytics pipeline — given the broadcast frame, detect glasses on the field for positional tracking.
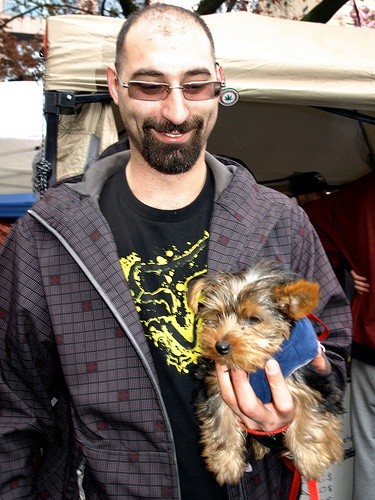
[116,62,222,101]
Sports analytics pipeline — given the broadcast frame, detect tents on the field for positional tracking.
[32,7,375,195]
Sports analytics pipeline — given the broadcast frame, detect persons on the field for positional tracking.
[0,2,351,500]
[314,181,375,500]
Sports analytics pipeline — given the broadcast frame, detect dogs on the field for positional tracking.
[186,258,345,488]
